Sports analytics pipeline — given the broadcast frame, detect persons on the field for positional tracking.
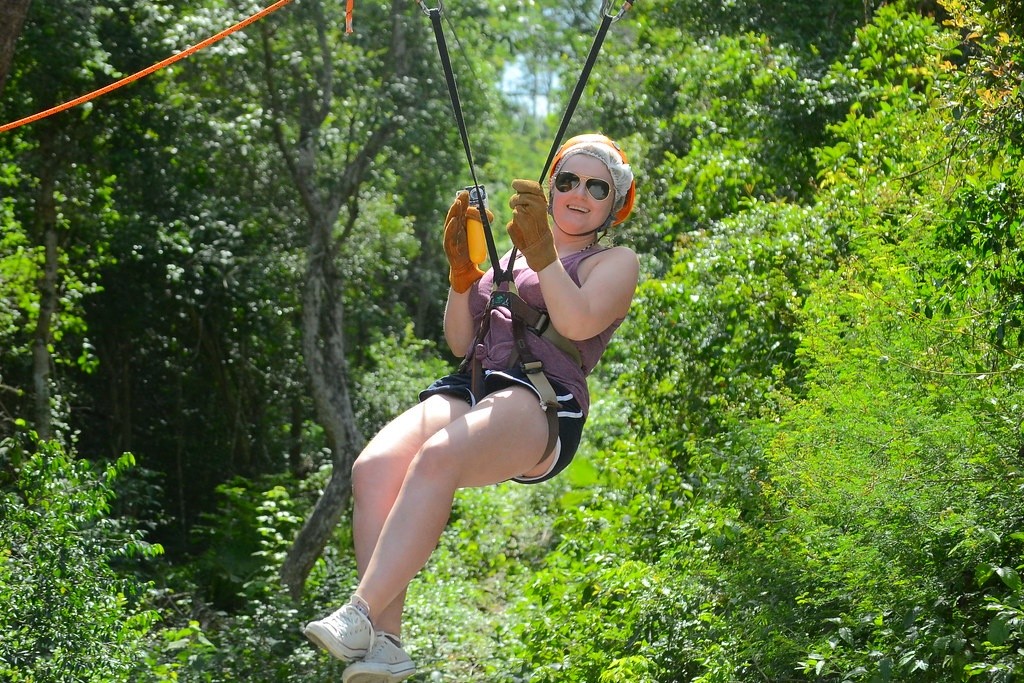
[304,133,639,682]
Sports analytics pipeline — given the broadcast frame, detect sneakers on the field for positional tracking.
[304,594,416,683]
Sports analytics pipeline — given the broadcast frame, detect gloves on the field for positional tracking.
[442,191,495,294]
[505,179,559,273]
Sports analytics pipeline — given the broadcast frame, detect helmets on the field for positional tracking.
[549,134,635,227]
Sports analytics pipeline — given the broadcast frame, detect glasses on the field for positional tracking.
[554,171,614,202]
